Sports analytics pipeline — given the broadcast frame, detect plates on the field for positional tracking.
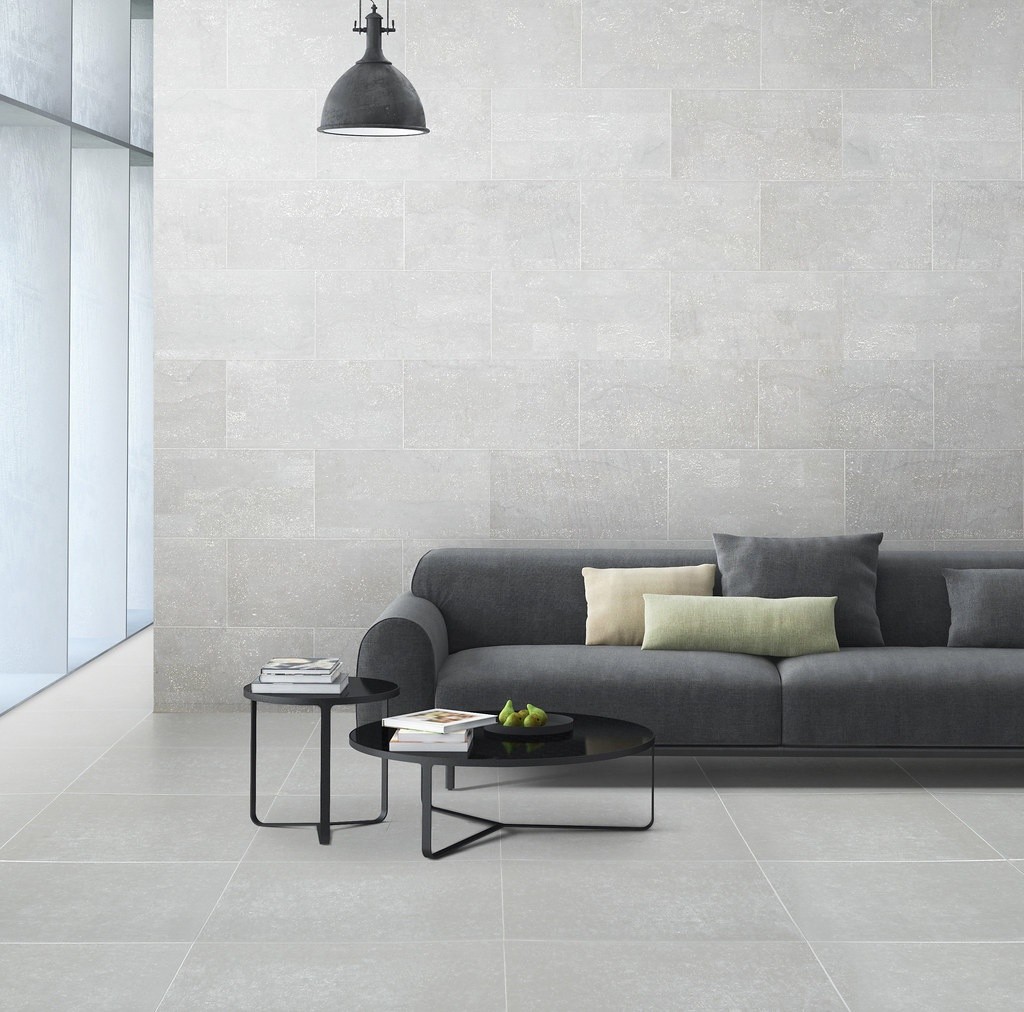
[485,713,573,733]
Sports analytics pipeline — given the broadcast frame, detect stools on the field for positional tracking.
[243,677,401,845]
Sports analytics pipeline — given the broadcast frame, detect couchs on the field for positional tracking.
[354,548,1024,790]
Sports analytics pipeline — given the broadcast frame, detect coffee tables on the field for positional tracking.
[349,711,655,859]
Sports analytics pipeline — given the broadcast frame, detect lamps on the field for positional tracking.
[317,0,430,136]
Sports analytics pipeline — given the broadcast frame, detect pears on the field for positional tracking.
[499,699,547,727]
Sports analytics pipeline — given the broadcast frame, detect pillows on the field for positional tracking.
[712,532,884,646]
[641,593,839,657]
[940,567,1024,647]
[582,563,717,646]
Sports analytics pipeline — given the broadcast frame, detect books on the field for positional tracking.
[251,657,349,694]
[382,708,497,752]
